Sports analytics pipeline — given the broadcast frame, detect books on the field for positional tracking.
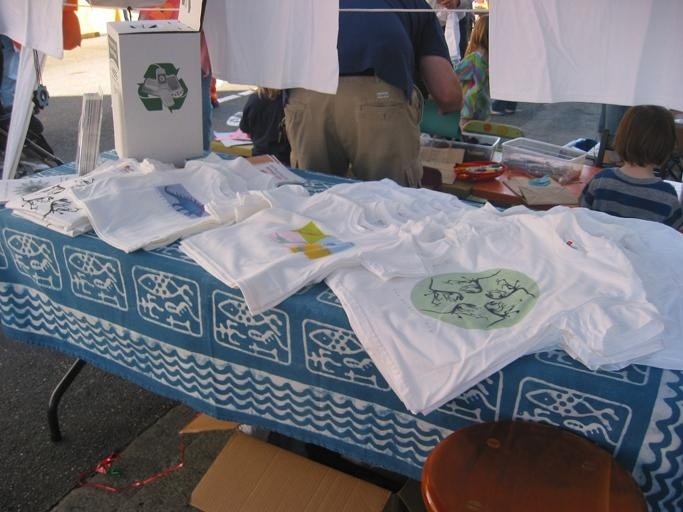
[210,140,254,157]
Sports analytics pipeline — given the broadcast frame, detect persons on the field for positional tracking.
[283,0,464,188]
[239,87,290,167]
[578,105,683,233]
[436,0,472,61]
[490,100,517,116]
[450,14,490,133]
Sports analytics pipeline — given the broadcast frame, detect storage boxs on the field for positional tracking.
[178,412,425,512]
[107,18,204,168]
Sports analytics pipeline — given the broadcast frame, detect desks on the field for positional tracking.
[407,130,683,222]
[211,129,261,168]
[2,153,682,512]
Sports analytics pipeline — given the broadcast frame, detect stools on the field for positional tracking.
[420,421,653,512]
